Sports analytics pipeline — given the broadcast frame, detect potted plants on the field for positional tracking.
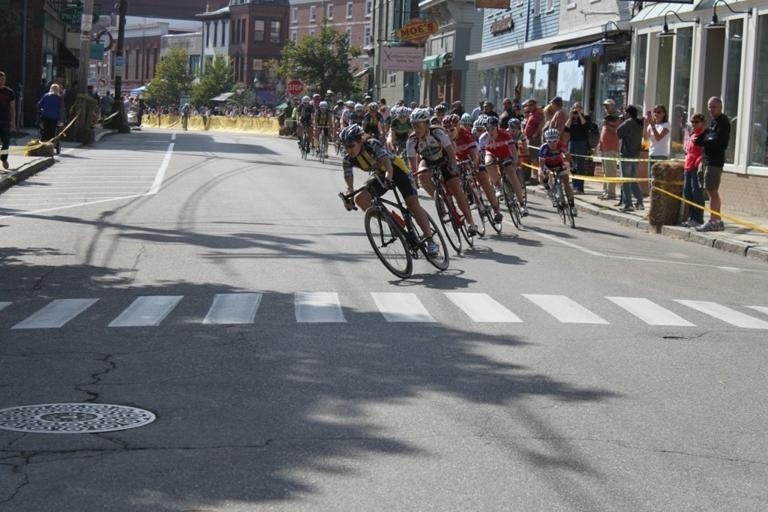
[636,141,653,196]
[672,140,685,159]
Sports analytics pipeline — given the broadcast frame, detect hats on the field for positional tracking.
[602,99,616,105]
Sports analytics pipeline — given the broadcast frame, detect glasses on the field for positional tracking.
[546,139,560,144]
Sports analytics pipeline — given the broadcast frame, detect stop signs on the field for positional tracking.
[287,81,303,95]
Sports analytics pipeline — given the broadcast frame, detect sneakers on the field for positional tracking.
[571,205,578,217]
[597,191,616,200]
[519,205,529,217]
[619,204,644,213]
[492,213,503,223]
[681,217,725,232]
[427,241,440,255]
[494,182,504,198]
[467,223,478,234]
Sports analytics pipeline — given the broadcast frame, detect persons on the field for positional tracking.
[36,78,280,150]
[0,71,17,169]
[288,90,731,259]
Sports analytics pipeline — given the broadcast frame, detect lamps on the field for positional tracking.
[705,0,753,29]
[597,20,632,45]
[656,10,700,37]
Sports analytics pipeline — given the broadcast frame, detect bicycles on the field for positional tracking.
[547,166,575,228]
[339,147,527,278]
[298,121,346,164]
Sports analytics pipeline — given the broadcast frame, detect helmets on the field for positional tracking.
[544,127,560,140]
[409,105,519,131]
[299,94,411,118]
[338,123,364,145]
